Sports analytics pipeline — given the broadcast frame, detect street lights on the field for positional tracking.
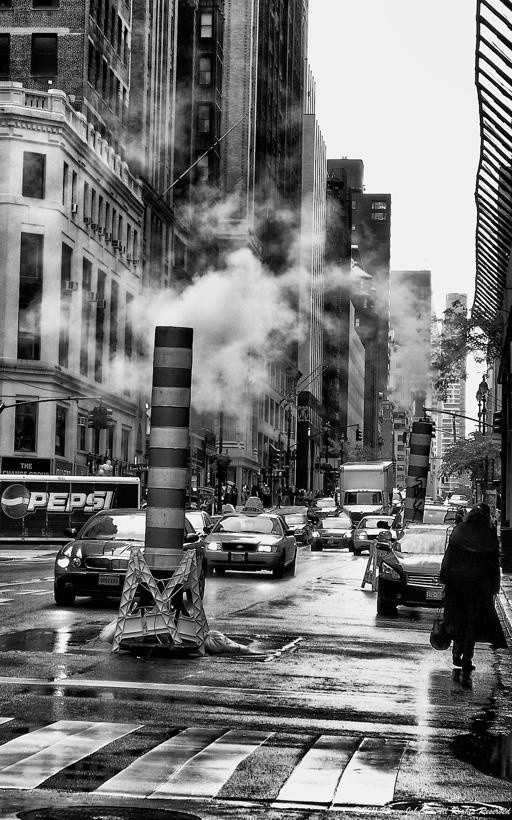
[474,387,483,434]
[480,374,488,436]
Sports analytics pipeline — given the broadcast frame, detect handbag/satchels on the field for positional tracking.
[429,617,451,650]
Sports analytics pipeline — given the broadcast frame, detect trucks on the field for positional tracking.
[337,459,395,523]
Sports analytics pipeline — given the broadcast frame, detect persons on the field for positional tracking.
[454,505,464,525]
[438,503,510,671]
[219,479,339,511]
[447,492,452,499]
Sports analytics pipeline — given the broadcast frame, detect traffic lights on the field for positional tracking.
[427,418,436,439]
[100,407,115,430]
[355,429,363,442]
[491,408,503,435]
[271,446,281,465]
[87,406,100,430]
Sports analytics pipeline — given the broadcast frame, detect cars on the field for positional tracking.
[179,490,469,575]
[50,505,209,613]
[366,521,457,620]
